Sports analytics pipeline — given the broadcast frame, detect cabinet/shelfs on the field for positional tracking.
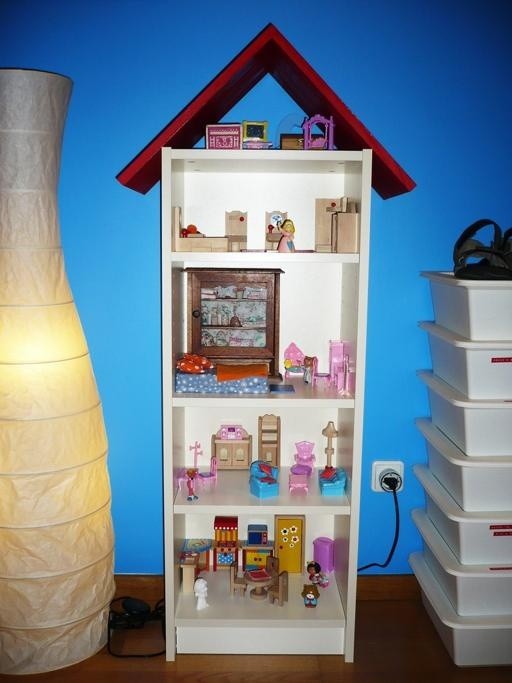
[158,143,375,664]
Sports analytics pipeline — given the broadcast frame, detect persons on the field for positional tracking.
[276,219,296,253]
[306,561,327,585]
[185,468,199,501]
[193,578,210,610]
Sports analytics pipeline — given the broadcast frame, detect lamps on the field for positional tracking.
[1,66,117,678]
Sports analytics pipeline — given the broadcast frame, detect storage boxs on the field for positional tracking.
[415,370,512,460]
[412,463,512,568]
[417,321,512,400]
[419,269,512,341]
[415,416,512,513]
[410,508,512,616]
[408,549,512,668]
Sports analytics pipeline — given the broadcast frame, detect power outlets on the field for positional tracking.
[369,457,405,493]
